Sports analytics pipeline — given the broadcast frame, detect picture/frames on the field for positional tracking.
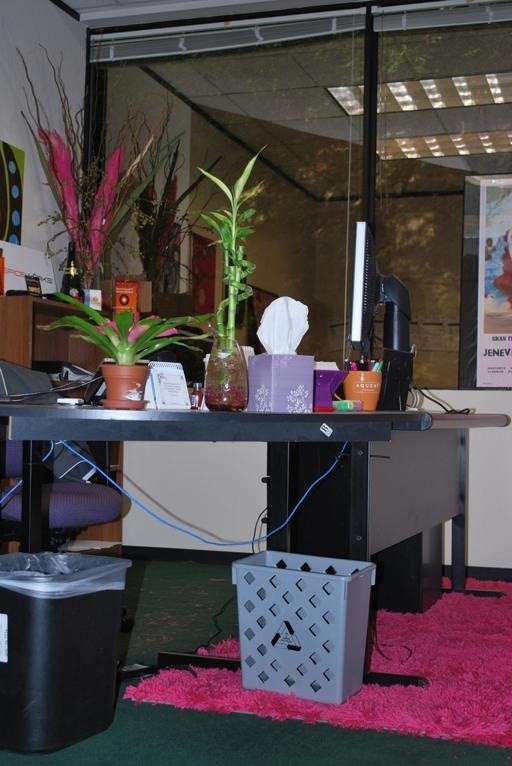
[458,172,512,392]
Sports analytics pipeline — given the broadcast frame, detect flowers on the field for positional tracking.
[14,31,185,299]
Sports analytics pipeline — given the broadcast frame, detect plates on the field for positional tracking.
[99,398,150,409]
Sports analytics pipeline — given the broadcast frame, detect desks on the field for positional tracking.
[0,403,510,684]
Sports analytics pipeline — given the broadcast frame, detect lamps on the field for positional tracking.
[325,71,512,117]
[375,129,512,161]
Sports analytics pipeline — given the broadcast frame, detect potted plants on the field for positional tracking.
[36,291,215,412]
[197,143,273,412]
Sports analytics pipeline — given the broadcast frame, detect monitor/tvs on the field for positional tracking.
[350,221,411,354]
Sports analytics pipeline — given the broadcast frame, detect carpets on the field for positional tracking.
[121,575,512,750]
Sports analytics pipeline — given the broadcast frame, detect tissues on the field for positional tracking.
[246,294,314,413]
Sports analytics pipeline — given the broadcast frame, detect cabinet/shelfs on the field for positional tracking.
[1,273,138,374]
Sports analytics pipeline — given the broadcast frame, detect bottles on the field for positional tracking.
[190,383,203,410]
[63,241,82,304]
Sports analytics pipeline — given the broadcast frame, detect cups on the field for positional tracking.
[344,371,384,411]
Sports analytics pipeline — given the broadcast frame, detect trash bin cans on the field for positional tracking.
[232,550,376,707]
[0,552,133,756]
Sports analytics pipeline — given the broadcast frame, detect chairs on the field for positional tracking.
[0,358,123,556]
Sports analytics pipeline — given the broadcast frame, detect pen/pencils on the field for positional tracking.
[344,355,384,371]
